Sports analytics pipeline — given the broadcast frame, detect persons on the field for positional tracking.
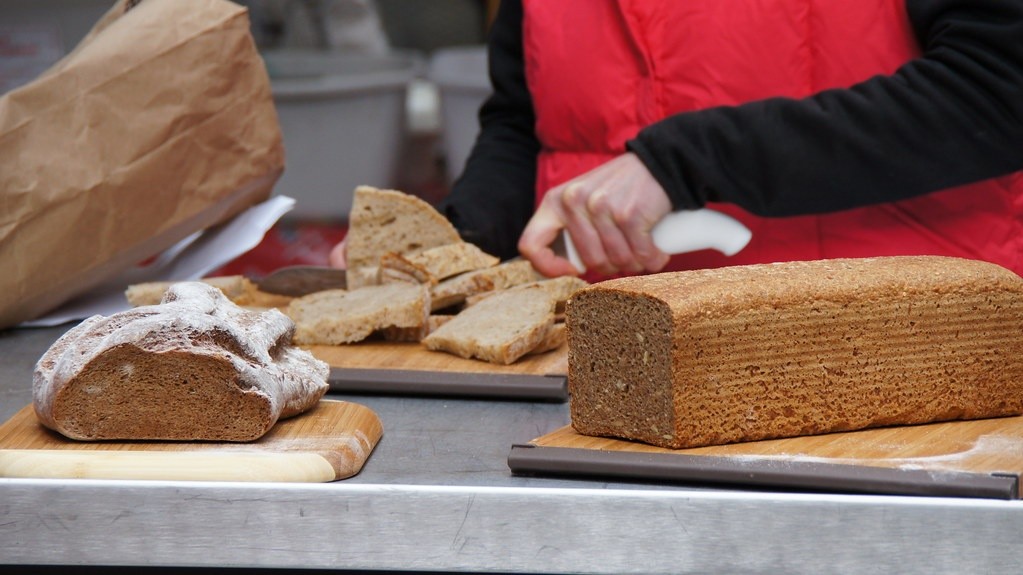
[324,0,1023,285]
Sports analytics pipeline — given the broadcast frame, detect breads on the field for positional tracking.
[32,184,1023,451]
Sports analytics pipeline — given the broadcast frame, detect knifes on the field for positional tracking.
[494,204,751,281]
